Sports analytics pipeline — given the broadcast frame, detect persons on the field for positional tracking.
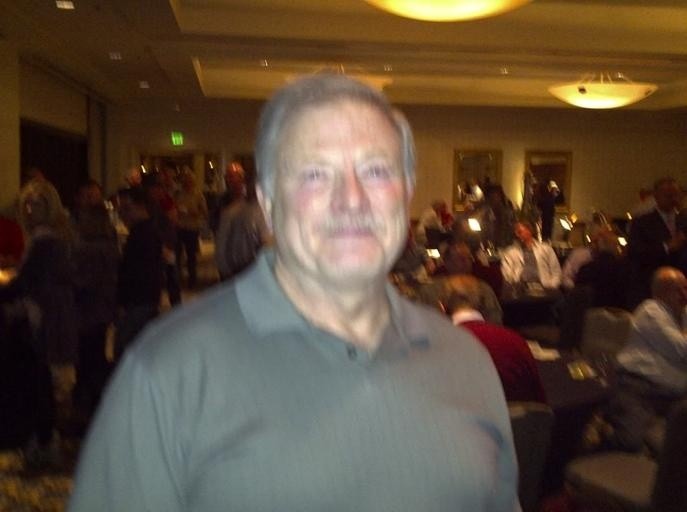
[0,134,265,478]
[385,163,686,512]
[65,65,525,512]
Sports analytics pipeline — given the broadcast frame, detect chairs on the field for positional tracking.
[562,402,686,511]
[507,398,555,511]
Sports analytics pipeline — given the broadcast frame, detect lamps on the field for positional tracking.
[548,70,656,110]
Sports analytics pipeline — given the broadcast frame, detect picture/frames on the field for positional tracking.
[525,149,571,214]
[452,149,503,214]
